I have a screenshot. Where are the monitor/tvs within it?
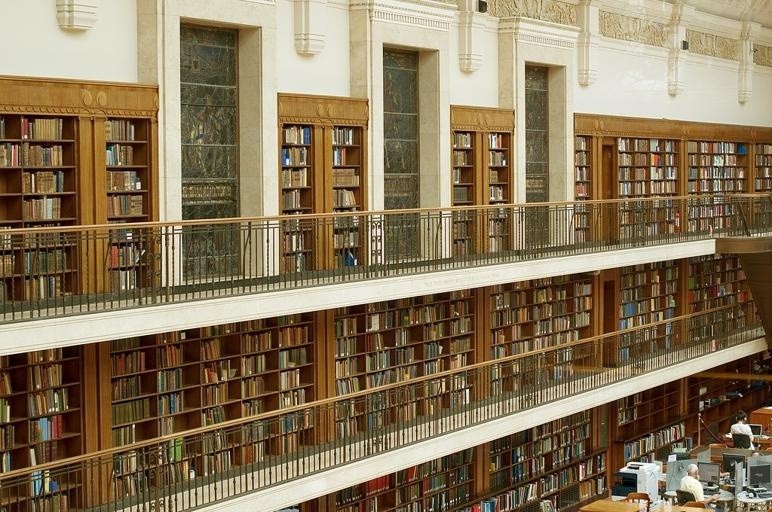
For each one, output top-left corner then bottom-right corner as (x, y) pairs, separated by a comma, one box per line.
(750, 464), (771, 485)
(611, 460), (657, 504)
(723, 453), (745, 479)
(697, 462), (720, 484)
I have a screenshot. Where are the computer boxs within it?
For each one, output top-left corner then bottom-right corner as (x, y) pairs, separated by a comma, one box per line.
(703, 486), (720, 494)
(691, 446), (711, 465)
(747, 487), (768, 497)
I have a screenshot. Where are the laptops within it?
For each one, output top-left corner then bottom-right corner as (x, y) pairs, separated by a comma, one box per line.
(749, 424), (762, 437)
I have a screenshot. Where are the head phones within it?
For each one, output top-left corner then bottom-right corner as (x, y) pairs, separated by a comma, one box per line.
(678, 463), (685, 471)
(735, 414), (747, 423)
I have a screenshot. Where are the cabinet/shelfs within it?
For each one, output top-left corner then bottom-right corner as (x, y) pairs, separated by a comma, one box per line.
(278, 107), (370, 276)
(574, 113), (772, 245)
(0, 109), (158, 313)
(1, 254), (771, 511)
(449, 105), (515, 258)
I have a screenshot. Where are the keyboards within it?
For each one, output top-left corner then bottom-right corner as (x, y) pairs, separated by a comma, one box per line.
(758, 493), (772, 498)
